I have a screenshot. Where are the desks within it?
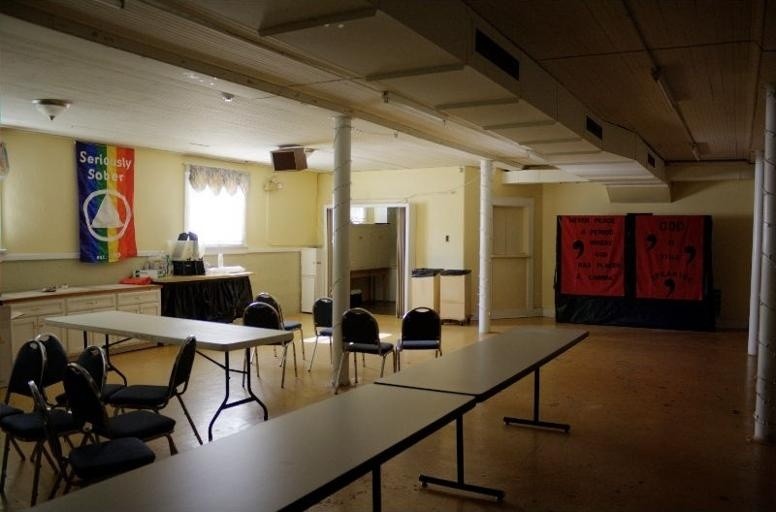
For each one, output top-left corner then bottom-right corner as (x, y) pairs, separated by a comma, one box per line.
(152, 271), (255, 324)
(348, 266), (391, 310)
(15, 384), (479, 512)
(373, 325), (589, 507)
(43, 310), (294, 443)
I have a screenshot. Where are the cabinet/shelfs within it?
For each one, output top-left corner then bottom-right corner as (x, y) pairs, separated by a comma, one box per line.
(0, 292), (67, 369)
(65, 287), (116, 360)
(115, 283), (163, 357)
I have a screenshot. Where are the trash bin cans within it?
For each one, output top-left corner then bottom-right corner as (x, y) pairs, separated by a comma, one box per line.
(350, 289), (362, 308)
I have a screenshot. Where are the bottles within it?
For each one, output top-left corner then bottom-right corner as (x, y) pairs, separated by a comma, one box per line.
(217, 253), (224, 269)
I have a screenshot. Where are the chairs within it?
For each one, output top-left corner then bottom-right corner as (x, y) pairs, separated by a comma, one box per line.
(331, 306), (396, 396)
(306, 297), (366, 374)
(2, 330), (200, 512)
(240, 301), (299, 389)
(250, 292), (306, 365)
(395, 306), (443, 373)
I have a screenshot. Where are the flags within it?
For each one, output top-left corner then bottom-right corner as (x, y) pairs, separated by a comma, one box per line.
(74, 141), (138, 264)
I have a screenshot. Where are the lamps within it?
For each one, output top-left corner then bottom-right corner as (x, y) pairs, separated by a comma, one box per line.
(31, 99), (69, 119)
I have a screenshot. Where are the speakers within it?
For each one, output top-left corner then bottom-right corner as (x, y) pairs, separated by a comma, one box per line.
(270, 147), (308, 171)
